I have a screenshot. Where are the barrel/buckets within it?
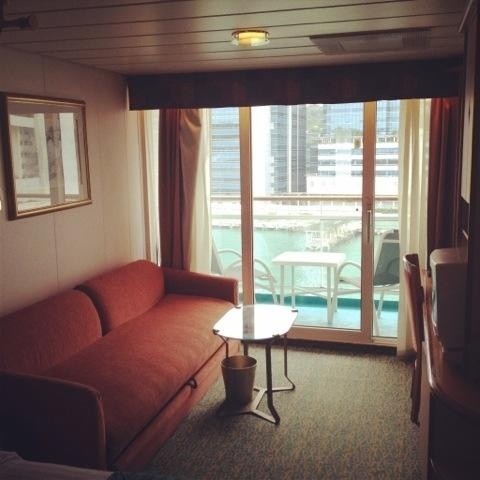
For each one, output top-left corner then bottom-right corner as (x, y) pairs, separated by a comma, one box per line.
(220, 355), (257, 403)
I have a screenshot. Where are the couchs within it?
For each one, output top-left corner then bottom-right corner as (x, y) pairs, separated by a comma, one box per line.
(0, 259), (242, 478)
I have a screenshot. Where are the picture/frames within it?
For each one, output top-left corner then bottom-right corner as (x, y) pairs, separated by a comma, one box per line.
(0, 92), (92, 221)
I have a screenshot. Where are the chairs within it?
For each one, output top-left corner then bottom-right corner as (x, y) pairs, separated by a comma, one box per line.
(331, 230), (401, 337)
(402, 254), (425, 424)
(218, 249), (278, 306)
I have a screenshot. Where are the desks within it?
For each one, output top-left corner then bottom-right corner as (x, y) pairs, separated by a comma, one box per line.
(271, 251), (346, 327)
(212, 303), (298, 424)
